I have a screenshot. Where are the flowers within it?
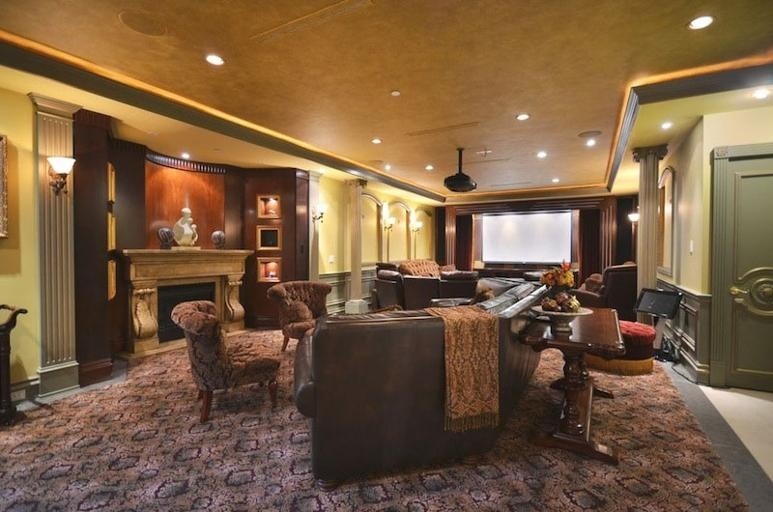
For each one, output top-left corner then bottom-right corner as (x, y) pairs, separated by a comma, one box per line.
(540, 259), (575, 288)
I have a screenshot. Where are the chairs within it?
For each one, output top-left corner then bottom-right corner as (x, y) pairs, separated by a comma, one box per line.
(266, 281), (332, 351)
(568, 261), (637, 322)
(170, 301), (282, 424)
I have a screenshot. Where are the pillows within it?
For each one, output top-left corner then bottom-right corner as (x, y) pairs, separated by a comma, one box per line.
(290, 300), (313, 322)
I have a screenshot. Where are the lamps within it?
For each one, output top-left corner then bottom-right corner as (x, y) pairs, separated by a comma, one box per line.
(412, 221), (423, 233)
(312, 205), (329, 223)
(628, 213), (640, 225)
(47, 157), (76, 195)
(384, 218), (396, 231)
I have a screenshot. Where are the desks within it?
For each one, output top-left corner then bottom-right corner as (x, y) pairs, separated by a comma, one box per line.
(520, 307), (627, 465)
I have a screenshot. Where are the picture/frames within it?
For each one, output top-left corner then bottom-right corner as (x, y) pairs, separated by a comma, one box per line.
(256, 192), (282, 282)
(0, 135), (8, 239)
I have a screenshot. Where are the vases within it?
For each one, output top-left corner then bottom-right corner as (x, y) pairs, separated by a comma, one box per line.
(551, 284), (566, 299)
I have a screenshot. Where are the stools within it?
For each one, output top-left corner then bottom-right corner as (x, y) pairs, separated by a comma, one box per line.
(584, 319), (656, 375)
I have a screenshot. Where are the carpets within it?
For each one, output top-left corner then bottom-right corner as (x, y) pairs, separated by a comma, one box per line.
(0, 328), (748, 512)
